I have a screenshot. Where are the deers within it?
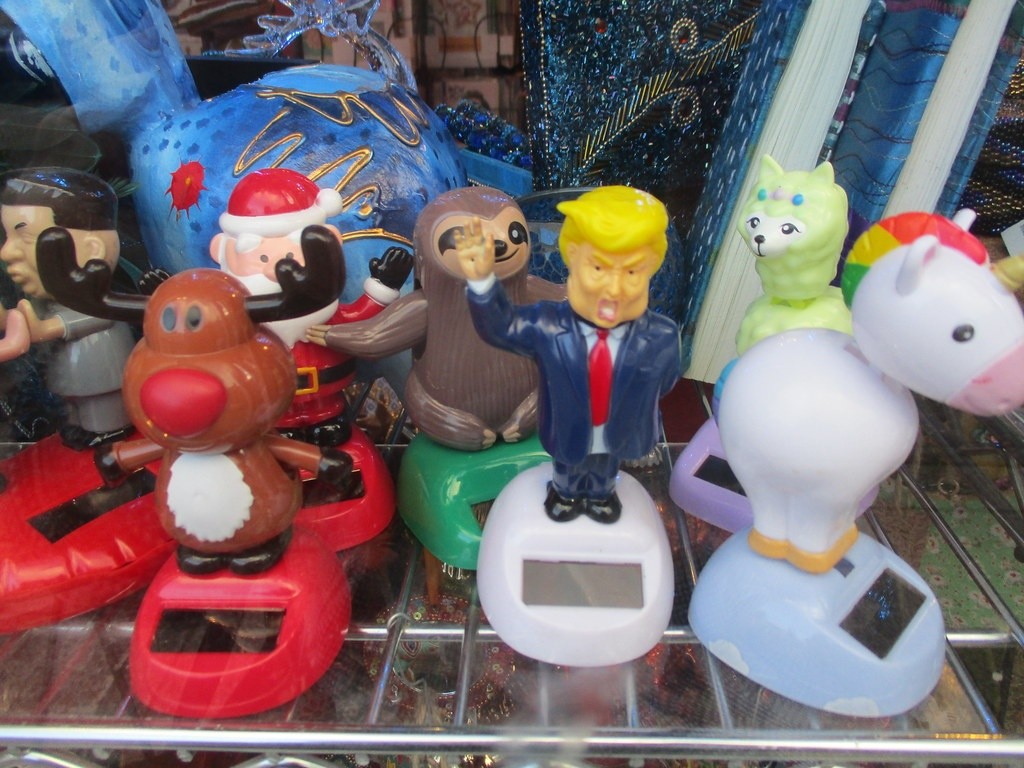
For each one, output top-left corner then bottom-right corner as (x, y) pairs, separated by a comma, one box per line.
(35, 224), (353, 571)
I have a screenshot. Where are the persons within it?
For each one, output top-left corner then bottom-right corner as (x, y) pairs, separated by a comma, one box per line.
(0, 165), (134, 450)
(210, 168), (413, 444)
(452, 186), (682, 525)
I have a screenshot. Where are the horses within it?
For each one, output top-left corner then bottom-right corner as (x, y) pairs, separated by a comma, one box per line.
(711, 208), (1024, 570)
